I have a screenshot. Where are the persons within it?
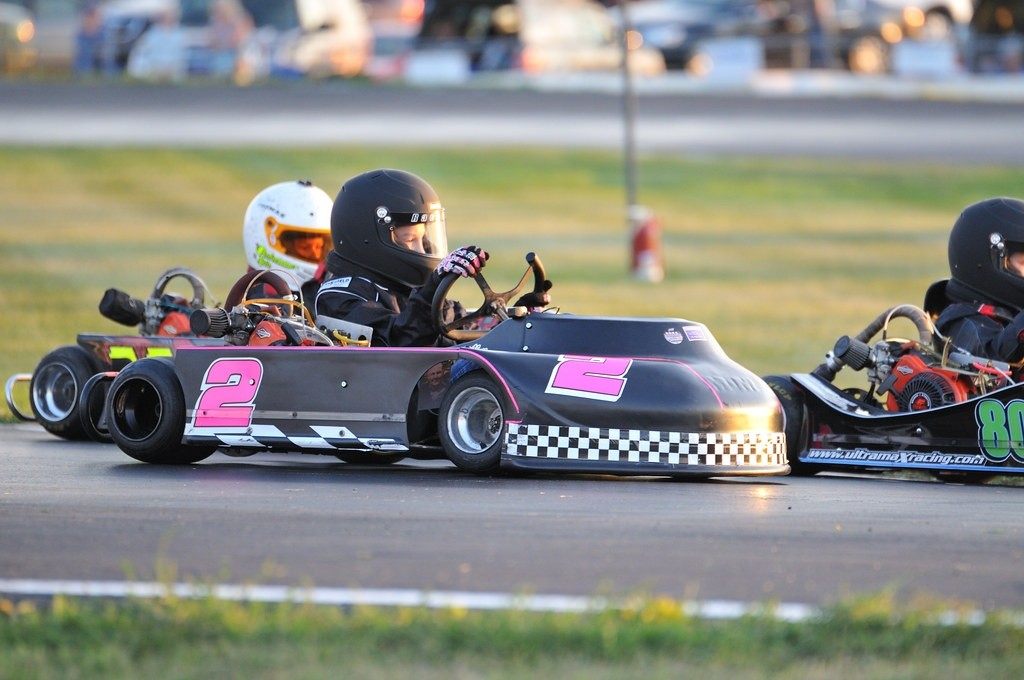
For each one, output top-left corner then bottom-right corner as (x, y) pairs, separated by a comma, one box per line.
(934, 198), (1024, 366)
(315, 169), (572, 347)
(225, 179), (332, 324)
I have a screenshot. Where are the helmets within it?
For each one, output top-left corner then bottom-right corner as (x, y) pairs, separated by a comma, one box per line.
(326, 168), (445, 287)
(243, 181), (333, 292)
(948, 198), (1024, 312)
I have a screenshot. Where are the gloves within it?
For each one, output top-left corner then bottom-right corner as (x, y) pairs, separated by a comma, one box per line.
(520, 279), (553, 313)
(427, 244), (491, 281)
